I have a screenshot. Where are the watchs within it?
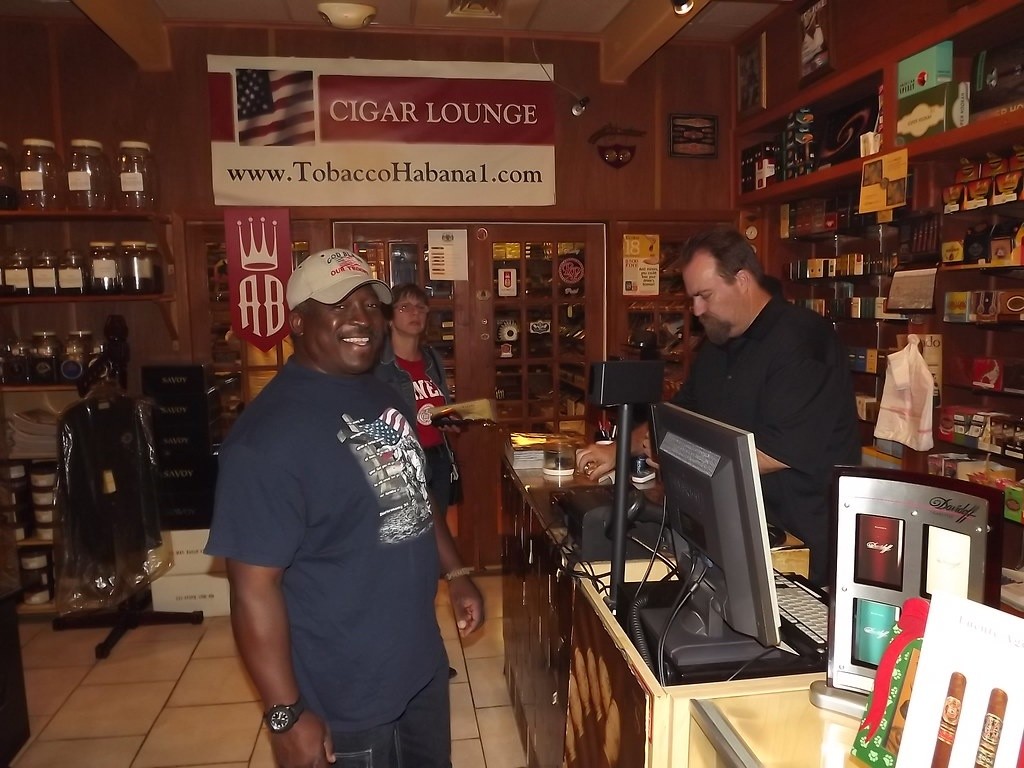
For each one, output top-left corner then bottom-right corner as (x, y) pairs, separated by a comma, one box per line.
(263, 696), (305, 734)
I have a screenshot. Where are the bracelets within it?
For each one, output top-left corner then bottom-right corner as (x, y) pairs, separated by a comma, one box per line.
(443, 567), (474, 581)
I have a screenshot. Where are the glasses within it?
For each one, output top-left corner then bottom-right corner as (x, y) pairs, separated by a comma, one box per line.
(400, 303), (430, 313)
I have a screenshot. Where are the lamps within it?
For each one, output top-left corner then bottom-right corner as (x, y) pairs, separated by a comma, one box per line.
(317, 3), (376, 31)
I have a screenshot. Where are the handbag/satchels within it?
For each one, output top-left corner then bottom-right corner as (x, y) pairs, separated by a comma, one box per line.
(442, 442), (463, 507)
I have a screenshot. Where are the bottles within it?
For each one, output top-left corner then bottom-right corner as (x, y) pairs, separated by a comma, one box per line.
(0, 142), (17, 210)
(29, 332), (63, 356)
(115, 141), (158, 211)
(119, 240), (152, 294)
(58, 250), (89, 295)
(5, 337), (30, 356)
(32, 250), (59, 294)
(146, 243), (165, 294)
(4, 246), (34, 295)
(65, 330), (94, 364)
(67, 139), (113, 210)
(87, 242), (122, 293)
(16, 138), (64, 210)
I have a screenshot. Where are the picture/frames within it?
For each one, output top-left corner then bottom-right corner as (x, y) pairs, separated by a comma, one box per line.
(735, 29), (768, 121)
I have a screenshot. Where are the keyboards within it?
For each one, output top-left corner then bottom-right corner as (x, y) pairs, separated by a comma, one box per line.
(771, 568), (830, 653)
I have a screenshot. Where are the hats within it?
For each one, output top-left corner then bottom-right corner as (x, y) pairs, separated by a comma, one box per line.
(287, 248), (394, 312)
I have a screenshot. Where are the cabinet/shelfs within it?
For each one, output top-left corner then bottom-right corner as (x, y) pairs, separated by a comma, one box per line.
(728, 1), (1023, 523)
(0, 208), (174, 611)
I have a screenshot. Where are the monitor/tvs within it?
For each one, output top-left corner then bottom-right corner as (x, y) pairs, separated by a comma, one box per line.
(638, 400), (780, 666)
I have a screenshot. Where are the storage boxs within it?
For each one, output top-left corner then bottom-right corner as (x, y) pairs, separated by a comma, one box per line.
(148, 529), (228, 576)
(155, 573), (230, 615)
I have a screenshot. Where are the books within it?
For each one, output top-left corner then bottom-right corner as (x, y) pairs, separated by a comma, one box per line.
(8, 408), (57, 458)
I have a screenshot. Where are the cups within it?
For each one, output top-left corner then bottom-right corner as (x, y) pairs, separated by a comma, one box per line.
(596, 440), (615, 445)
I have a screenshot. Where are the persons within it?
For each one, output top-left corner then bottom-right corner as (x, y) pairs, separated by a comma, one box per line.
(576, 223), (861, 593)
(202, 248), (485, 768)
(381, 283), (463, 519)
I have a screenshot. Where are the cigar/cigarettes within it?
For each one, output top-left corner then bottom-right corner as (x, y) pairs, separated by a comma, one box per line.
(931, 672), (967, 768)
(974, 688), (1008, 768)
